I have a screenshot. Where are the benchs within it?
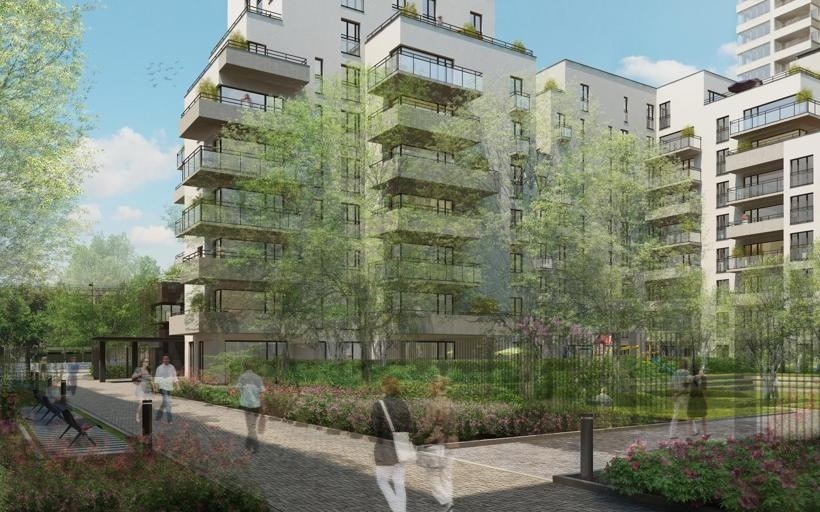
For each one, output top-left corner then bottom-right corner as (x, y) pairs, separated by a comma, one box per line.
(40, 395), (69, 426)
(59, 408), (104, 447)
(31, 388), (47, 414)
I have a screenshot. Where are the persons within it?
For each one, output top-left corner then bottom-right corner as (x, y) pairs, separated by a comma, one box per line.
(669, 359), (702, 440)
(367, 377), (411, 511)
(413, 374), (456, 509)
(67, 357), (80, 396)
(673, 363), (712, 439)
(154, 355), (180, 427)
(132, 357), (155, 425)
(237, 364), (265, 456)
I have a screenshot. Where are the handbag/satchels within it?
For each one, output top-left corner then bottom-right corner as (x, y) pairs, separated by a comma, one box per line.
(391, 431), (417, 465)
(258, 412), (266, 434)
(415, 443), (446, 468)
